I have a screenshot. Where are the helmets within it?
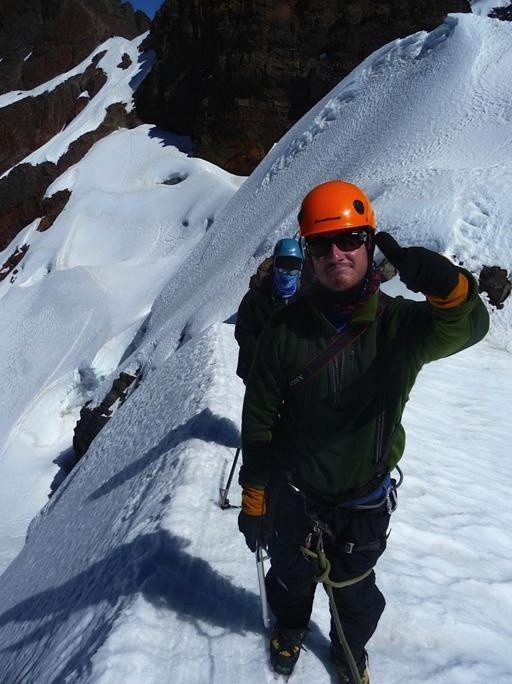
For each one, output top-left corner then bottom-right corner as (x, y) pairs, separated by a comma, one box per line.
(273, 238), (304, 261)
(298, 181), (376, 236)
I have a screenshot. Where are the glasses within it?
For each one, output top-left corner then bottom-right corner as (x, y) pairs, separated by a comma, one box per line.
(309, 231), (367, 256)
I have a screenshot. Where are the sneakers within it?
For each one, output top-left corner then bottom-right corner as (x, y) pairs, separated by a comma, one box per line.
(270, 624), (307, 675)
(330, 646), (369, 684)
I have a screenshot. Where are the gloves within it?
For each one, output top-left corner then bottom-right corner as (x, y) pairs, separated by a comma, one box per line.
(375, 230), (458, 298)
(237, 489), (277, 552)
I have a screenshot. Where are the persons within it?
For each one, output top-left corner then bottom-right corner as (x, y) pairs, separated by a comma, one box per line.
(234, 178), (491, 684)
(235, 237), (304, 384)
